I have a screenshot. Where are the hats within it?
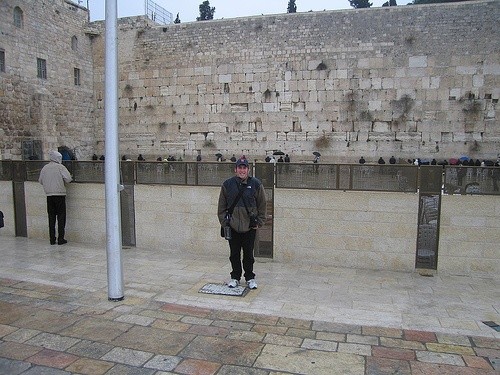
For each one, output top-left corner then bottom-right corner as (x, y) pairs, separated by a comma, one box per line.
(236, 158), (248, 167)
(240, 155), (246, 159)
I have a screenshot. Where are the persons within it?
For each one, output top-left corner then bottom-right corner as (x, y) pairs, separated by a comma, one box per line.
(278, 157), (283, 162)
(196, 152), (237, 162)
(217, 159), (267, 289)
(265, 156), (276, 166)
(407, 154), (500, 165)
(359, 156), (365, 164)
(390, 156), (396, 164)
(314, 155), (319, 163)
(378, 157), (385, 164)
(38, 151), (73, 245)
(92, 153), (183, 161)
(285, 154), (290, 162)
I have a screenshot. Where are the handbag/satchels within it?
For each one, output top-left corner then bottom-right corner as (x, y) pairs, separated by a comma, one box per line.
(221, 216), (230, 237)
(249, 216), (259, 229)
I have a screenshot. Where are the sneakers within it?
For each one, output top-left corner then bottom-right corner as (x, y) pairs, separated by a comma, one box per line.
(247, 279), (258, 289)
(228, 279), (240, 288)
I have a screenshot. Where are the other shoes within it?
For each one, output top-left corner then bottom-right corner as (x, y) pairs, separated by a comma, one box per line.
(50, 240), (56, 245)
(58, 239), (67, 245)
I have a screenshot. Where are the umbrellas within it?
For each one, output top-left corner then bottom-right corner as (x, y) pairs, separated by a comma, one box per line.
(313, 152), (321, 156)
(216, 153), (223, 157)
(273, 152), (285, 155)
